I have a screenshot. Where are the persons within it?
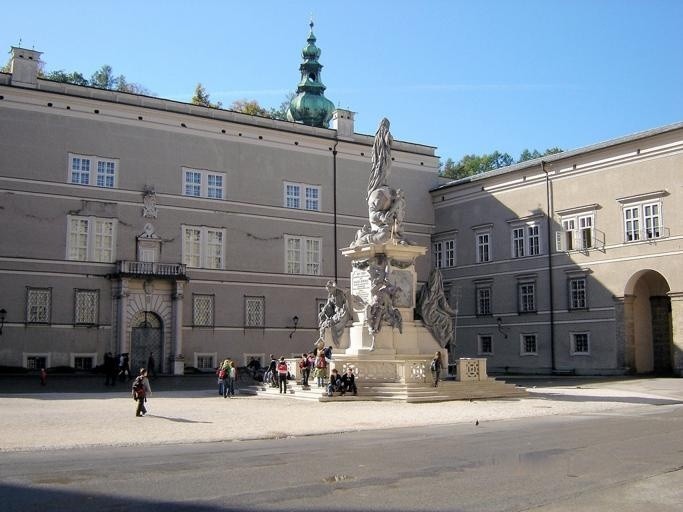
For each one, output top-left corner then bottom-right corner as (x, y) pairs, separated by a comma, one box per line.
(246, 354), (288, 394)
(105, 352), (132, 386)
(434, 351), (443, 387)
(147, 353), (158, 380)
(328, 368), (355, 396)
(41, 368), (46, 385)
(318, 117), (456, 346)
(216, 360), (237, 398)
(136, 368), (152, 416)
(300, 347), (333, 387)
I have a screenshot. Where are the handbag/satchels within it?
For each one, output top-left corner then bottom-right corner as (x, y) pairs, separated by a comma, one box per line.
(218, 379), (224, 384)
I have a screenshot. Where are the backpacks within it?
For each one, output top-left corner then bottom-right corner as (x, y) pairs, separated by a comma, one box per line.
(431, 359), (440, 371)
(132, 376), (146, 399)
(219, 368), (229, 379)
(278, 361), (287, 371)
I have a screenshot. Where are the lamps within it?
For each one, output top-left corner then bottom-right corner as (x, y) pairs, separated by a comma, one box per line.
(287, 316), (299, 337)
(0, 308), (6, 333)
(496, 316), (508, 341)
